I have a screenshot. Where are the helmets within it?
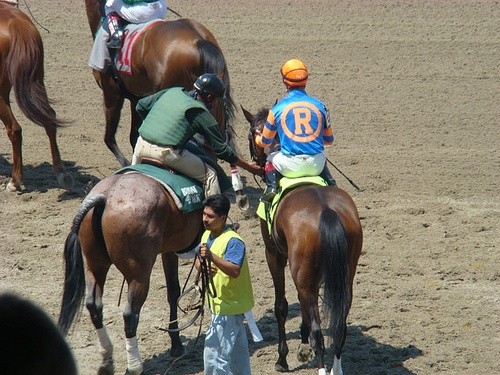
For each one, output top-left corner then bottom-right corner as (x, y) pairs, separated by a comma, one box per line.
(280, 59), (309, 87)
(193, 73), (225, 99)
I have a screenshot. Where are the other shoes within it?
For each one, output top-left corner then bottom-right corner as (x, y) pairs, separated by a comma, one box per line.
(227, 221), (240, 232)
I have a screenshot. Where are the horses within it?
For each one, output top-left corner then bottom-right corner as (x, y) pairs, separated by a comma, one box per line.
(238, 100), (363, 375)
(0, 0), (73, 192)
(58, 97), (232, 375)
(85, 1), (250, 211)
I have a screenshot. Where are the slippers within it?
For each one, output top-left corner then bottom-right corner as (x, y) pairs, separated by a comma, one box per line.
(318, 161), (336, 186)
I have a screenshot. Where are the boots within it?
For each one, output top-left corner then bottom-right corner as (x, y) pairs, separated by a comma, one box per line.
(261, 169), (280, 200)
(105, 11), (124, 49)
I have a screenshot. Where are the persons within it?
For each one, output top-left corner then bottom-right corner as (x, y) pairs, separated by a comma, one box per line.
(131, 74), (265, 230)
(195, 194), (254, 375)
(256, 58), (337, 204)
(89, 0), (167, 73)
